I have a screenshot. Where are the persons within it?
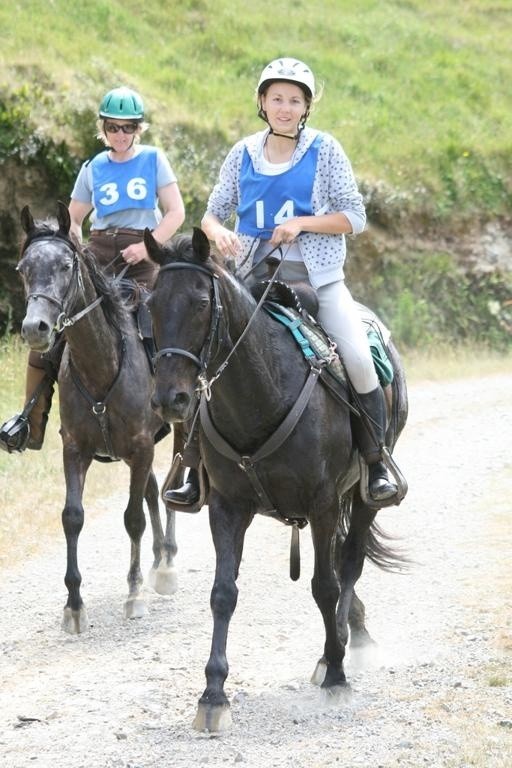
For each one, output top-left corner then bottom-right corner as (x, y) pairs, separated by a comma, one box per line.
(163, 57), (397, 505)
(12, 87), (185, 449)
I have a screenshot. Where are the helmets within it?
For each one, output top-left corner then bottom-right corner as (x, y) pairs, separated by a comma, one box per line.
(99, 87), (144, 119)
(256, 57), (314, 109)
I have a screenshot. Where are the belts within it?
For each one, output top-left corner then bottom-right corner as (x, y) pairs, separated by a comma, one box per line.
(91, 229), (143, 235)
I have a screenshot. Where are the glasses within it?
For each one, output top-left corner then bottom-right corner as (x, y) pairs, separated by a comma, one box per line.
(104, 123), (136, 134)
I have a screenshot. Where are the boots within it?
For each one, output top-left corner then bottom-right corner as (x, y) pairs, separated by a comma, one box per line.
(162, 399), (210, 504)
(356, 384), (398, 501)
(1, 365), (54, 450)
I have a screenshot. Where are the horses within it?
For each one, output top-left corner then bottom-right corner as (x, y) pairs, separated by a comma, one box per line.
(0, 200), (184, 635)
(143, 227), (408, 733)
